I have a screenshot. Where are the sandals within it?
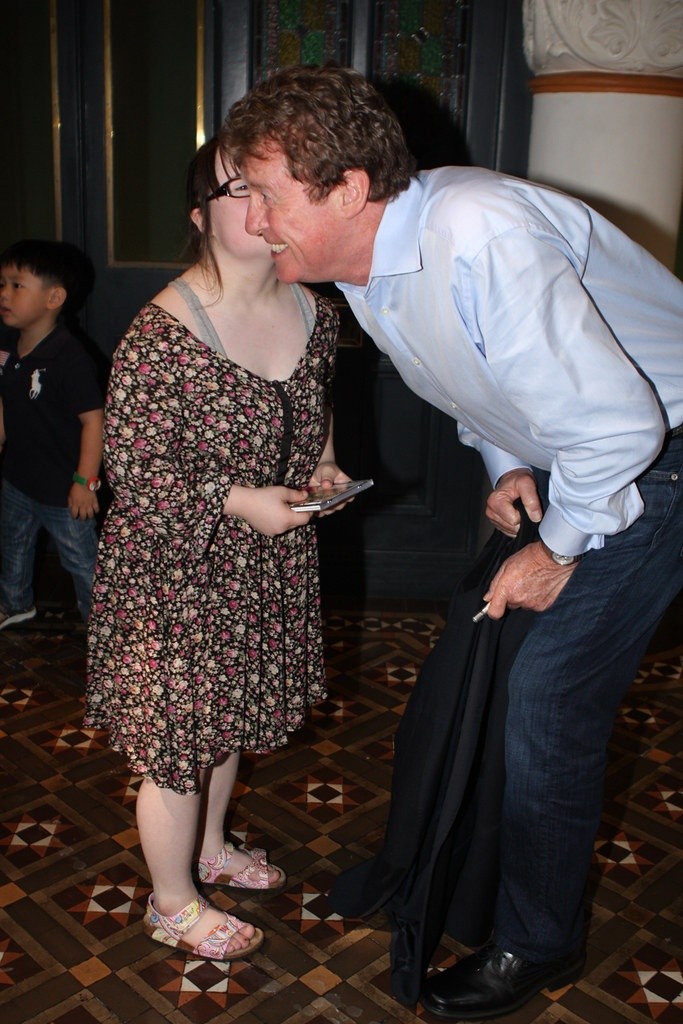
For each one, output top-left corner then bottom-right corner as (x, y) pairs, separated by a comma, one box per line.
(143, 893), (264, 960)
(0, 603), (36, 629)
(197, 842), (286, 889)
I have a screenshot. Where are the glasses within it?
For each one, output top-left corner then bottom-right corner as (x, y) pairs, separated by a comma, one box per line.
(205, 177), (251, 198)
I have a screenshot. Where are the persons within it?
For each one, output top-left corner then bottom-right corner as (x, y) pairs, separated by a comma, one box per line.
(81, 131), (356, 961)
(0, 241), (104, 630)
(219, 62), (683, 1024)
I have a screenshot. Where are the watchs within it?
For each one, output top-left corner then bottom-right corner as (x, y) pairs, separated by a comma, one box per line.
(540, 536), (587, 566)
(72, 472), (101, 492)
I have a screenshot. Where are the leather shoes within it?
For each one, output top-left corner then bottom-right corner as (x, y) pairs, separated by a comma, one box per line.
(420, 938), (586, 1022)
